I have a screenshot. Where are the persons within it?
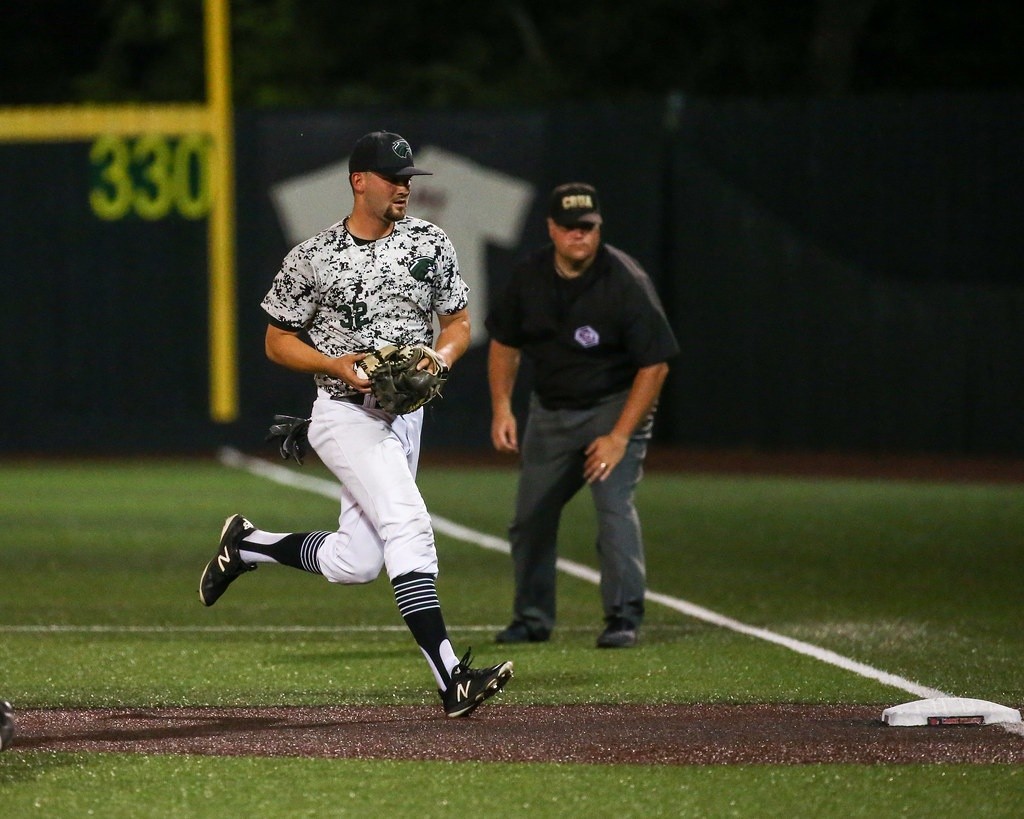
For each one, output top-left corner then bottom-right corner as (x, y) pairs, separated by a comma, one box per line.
(484, 183), (678, 647)
(198, 130), (514, 718)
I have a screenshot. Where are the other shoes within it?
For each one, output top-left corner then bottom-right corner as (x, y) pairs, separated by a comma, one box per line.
(496, 621), (551, 643)
(598, 616), (637, 647)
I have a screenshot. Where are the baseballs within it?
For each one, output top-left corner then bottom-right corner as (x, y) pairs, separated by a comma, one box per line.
(357, 366), (369, 380)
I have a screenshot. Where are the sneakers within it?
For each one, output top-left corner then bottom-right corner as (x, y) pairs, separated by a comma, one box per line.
(197, 514), (258, 607)
(438, 647), (514, 718)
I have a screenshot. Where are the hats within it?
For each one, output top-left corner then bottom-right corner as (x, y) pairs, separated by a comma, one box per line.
(349, 130), (434, 176)
(548, 184), (602, 228)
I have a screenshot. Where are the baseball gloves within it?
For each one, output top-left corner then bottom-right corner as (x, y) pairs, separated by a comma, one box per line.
(359, 343), (451, 417)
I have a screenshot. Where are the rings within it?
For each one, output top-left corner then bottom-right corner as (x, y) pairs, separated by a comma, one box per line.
(601, 462), (606, 468)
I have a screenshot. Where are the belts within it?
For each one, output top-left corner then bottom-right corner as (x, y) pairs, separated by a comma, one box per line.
(330, 393), (381, 408)
